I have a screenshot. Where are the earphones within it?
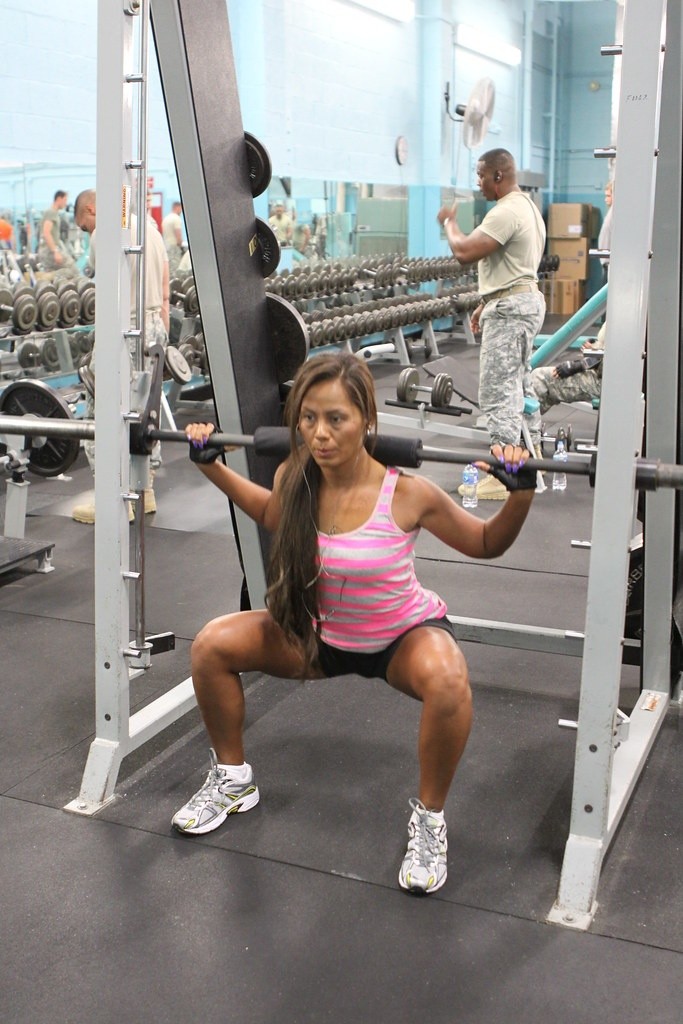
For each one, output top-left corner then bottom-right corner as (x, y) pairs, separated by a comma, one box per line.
(494, 174), (502, 184)
(366, 423), (371, 436)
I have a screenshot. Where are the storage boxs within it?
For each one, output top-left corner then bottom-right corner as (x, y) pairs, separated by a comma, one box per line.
(546, 237), (594, 281)
(537, 277), (587, 315)
(546, 201), (601, 241)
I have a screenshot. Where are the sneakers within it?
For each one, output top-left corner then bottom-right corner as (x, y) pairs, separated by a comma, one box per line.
(171, 764), (260, 834)
(127, 489), (157, 514)
(458, 474), (510, 500)
(73, 499), (134, 525)
(398, 804), (447, 893)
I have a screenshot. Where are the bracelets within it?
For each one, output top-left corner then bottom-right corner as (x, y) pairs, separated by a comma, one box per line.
(442, 217), (452, 226)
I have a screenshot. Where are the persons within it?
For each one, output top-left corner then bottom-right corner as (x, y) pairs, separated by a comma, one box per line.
(433, 150), (547, 500)
(596, 179), (609, 327)
(135, 190), (193, 270)
(518, 322), (605, 456)
(266, 201), (327, 263)
(2, 189), (74, 270)
(166, 353), (540, 893)
(73, 188), (162, 525)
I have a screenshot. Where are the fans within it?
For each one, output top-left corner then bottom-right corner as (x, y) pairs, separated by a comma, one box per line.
(456, 77), (496, 152)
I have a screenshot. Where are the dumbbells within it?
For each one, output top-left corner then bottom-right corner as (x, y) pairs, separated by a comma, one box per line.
(396, 368), (453, 409)
(540, 422), (574, 454)
(1, 253), (561, 386)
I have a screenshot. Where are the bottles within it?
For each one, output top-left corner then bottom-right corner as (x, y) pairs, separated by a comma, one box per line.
(551, 440), (567, 490)
(463, 462), (478, 508)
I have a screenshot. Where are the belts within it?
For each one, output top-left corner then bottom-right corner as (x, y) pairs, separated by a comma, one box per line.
(483, 284), (538, 303)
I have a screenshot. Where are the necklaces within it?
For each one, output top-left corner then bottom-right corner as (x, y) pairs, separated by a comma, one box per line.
(322, 445), (362, 535)
